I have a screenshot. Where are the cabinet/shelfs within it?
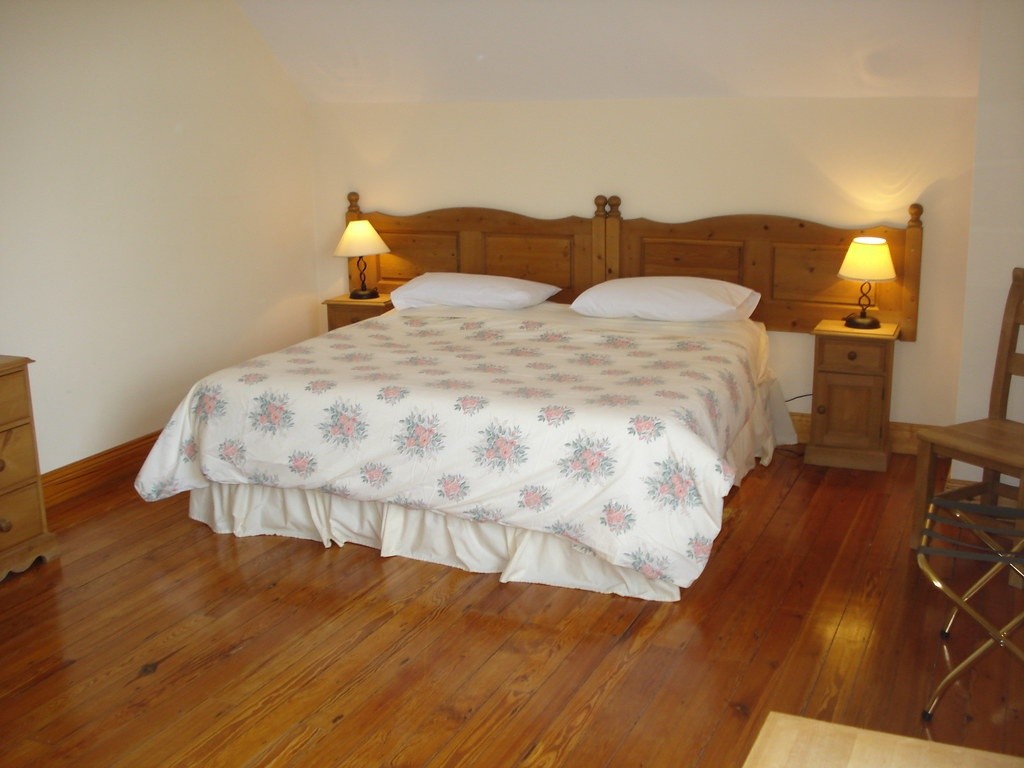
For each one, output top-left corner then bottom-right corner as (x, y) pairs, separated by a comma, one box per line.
(0, 354), (55, 585)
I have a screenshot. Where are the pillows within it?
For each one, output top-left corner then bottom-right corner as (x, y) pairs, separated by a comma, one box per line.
(571, 277), (762, 325)
(390, 271), (562, 311)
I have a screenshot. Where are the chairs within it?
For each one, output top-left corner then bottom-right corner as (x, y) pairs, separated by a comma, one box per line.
(905, 262), (1024, 590)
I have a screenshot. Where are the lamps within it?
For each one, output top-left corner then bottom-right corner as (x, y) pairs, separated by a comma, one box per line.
(334, 219), (390, 302)
(835, 237), (897, 331)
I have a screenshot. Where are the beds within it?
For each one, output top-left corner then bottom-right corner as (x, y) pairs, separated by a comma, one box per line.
(133, 184), (924, 603)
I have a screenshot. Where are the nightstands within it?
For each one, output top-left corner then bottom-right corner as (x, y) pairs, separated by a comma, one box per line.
(802, 317), (900, 474)
(322, 291), (393, 336)
